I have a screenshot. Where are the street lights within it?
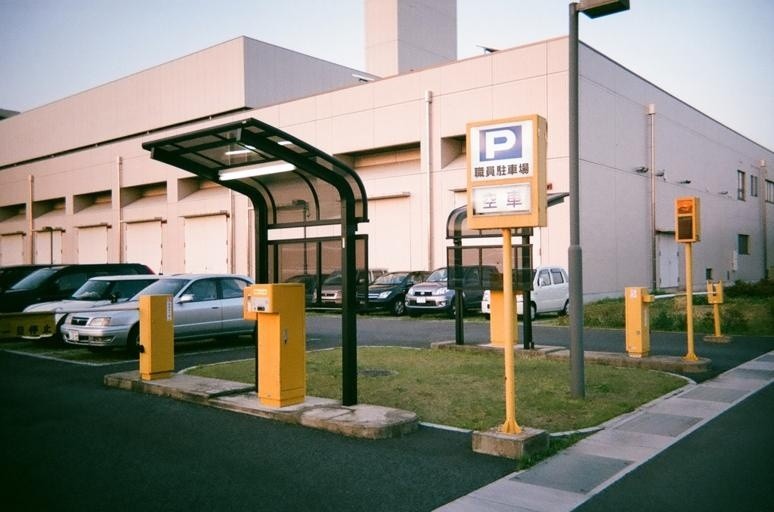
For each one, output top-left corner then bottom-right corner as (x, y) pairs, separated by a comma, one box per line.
(568, 0), (630, 398)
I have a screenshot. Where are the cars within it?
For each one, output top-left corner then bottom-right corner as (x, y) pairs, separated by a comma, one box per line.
(284, 265), (568, 321)
(1, 264), (256, 359)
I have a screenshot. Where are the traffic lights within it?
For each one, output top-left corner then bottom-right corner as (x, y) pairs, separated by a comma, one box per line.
(674, 197), (700, 243)
